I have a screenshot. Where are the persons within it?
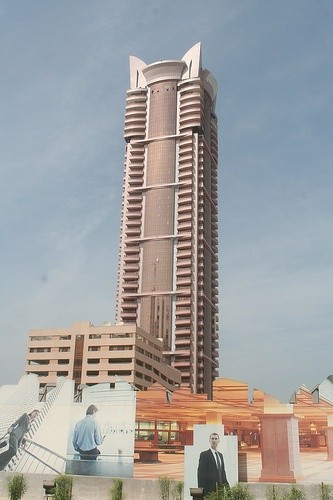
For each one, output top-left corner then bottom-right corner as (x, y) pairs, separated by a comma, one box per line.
(0, 410), (39, 471)
(197, 433), (231, 498)
(73, 404), (104, 460)
